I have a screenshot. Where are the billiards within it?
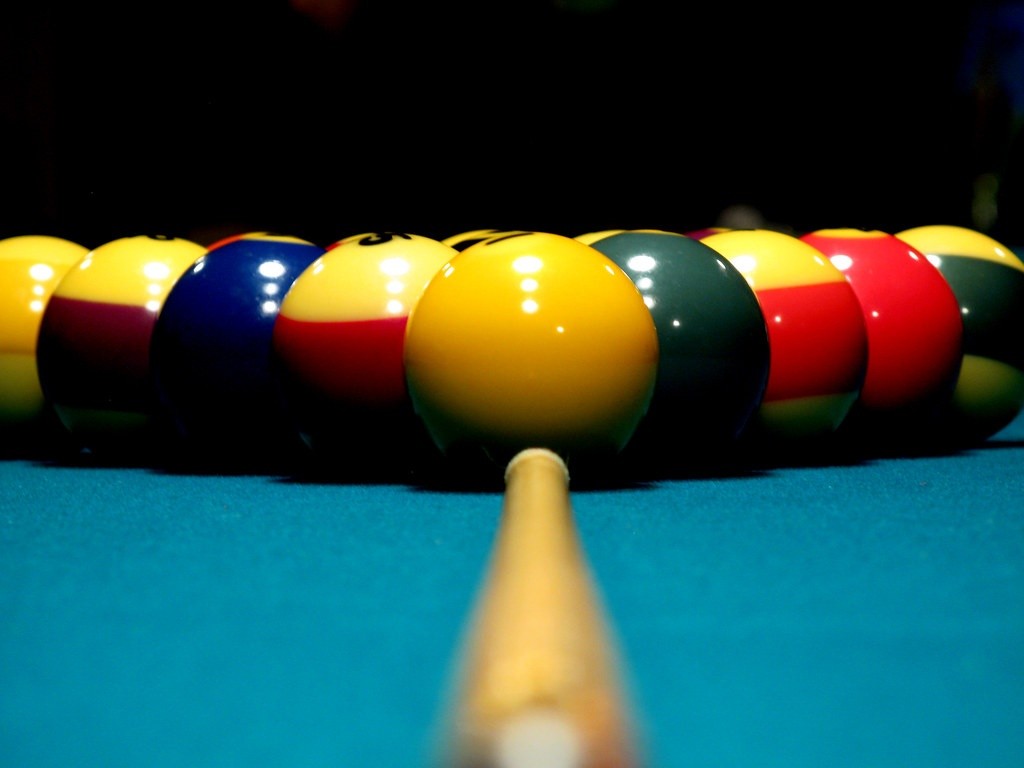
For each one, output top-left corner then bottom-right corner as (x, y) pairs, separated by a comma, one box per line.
(0, 224), (1022, 478)
(401, 230), (662, 488)
